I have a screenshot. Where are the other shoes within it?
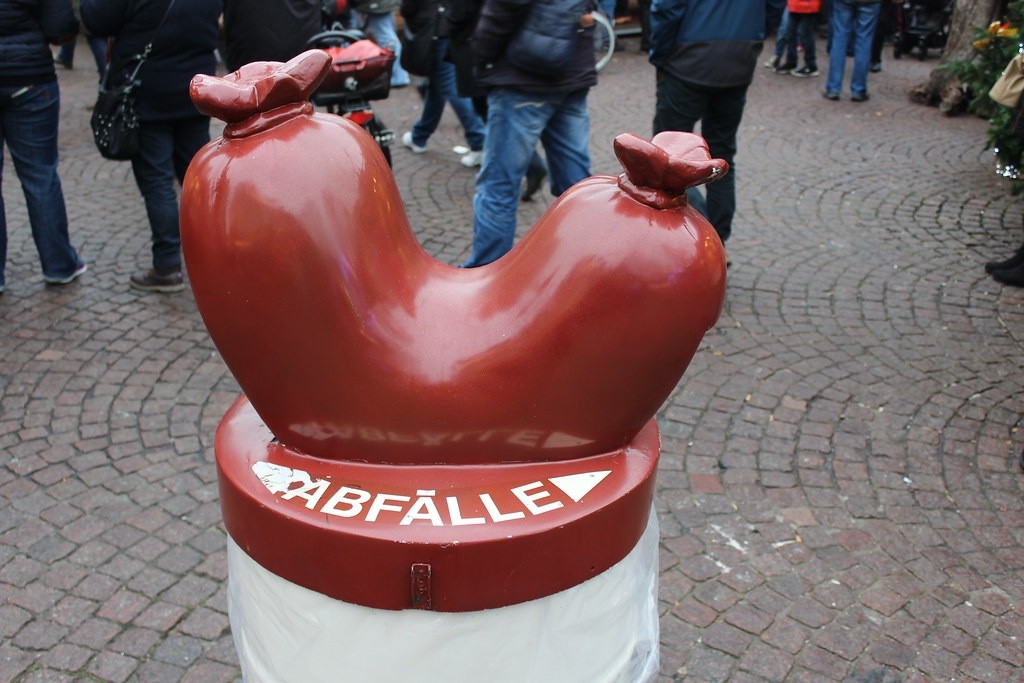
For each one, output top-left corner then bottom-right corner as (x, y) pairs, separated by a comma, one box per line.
(852, 92), (868, 101)
(821, 85), (838, 99)
(776, 61), (796, 74)
(523, 163), (548, 201)
(765, 53), (781, 69)
(872, 62), (880, 71)
(792, 65), (821, 78)
(53, 53), (72, 70)
(402, 129), (425, 152)
(602, 41), (626, 53)
(984, 243), (1024, 285)
(44, 263), (86, 284)
(460, 152), (485, 167)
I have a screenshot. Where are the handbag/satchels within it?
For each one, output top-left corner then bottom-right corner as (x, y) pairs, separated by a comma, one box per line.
(398, 22), (437, 77)
(988, 54), (1024, 106)
(89, 72), (137, 159)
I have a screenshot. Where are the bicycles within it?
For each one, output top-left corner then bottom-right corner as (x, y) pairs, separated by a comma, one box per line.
(305, 15), (396, 169)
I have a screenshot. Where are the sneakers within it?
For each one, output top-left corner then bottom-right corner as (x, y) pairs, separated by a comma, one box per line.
(129, 269), (183, 294)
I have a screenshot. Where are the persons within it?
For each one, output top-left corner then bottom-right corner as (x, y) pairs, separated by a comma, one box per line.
(0, 0), (889, 292)
(984, 48), (1024, 288)
